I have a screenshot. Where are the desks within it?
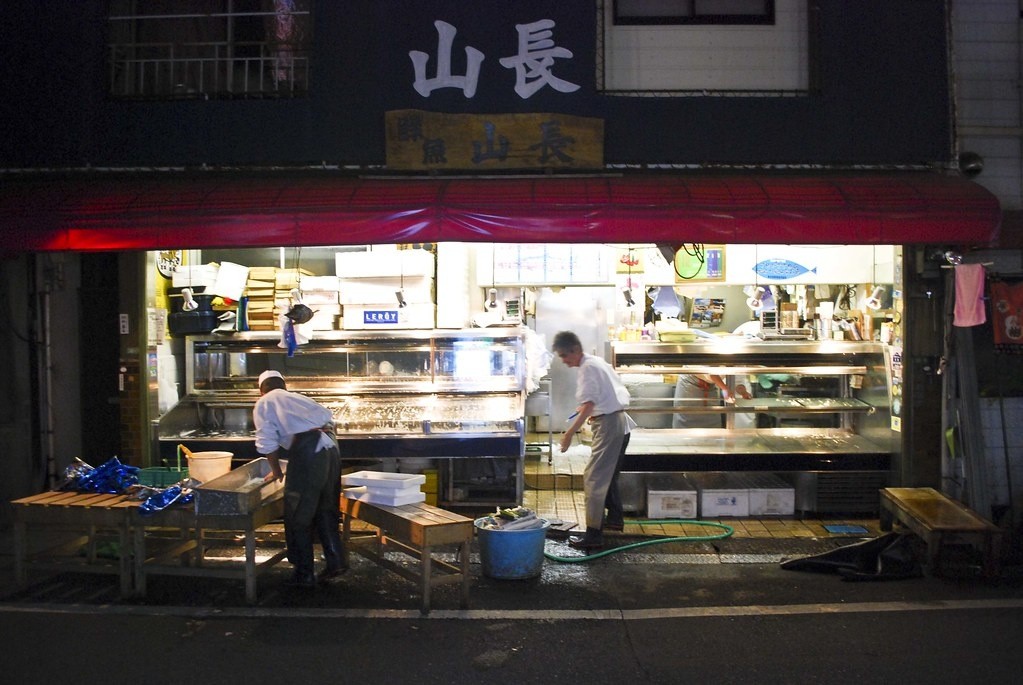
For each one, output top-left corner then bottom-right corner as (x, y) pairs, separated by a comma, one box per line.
(13, 469), (191, 600)
(334, 493), (474, 616)
(130, 492), (291, 604)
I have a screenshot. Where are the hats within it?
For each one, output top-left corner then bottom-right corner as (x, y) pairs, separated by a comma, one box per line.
(259, 370), (285, 388)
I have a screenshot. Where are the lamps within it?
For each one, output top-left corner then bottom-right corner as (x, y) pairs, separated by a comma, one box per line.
(622, 248), (641, 312)
(181, 253), (197, 312)
(865, 248), (886, 308)
(486, 242), (504, 313)
(746, 246), (766, 309)
(293, 251), (306, 308)
(395, 247), (409, 322)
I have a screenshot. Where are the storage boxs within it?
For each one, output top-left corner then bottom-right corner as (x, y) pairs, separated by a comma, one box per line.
(647, 473), (795, 520)
(246, 247), (436, 329)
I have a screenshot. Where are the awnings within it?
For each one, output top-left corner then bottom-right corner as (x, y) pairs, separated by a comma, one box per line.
(0, 172), (1005, 253)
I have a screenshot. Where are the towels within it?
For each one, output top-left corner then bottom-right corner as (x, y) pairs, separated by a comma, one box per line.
(954, 264), (986, 327)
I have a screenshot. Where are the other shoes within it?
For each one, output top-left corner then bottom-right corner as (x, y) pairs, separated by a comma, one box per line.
(604, 511), (624, 529)
(569, 526), (604, 547)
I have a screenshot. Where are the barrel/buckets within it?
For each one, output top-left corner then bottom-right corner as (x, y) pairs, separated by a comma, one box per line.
(474, 516), (551, 580)
(186, 451), (234, 484)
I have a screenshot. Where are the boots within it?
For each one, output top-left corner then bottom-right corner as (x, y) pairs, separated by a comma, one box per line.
(317, 520), (347, 579)
(283, 542), (318, 599)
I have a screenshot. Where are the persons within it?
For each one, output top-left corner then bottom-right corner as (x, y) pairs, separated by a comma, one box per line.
(253, 370), (348, 584)
(552, 330), (637, 549)
(672, 332), (753, 429)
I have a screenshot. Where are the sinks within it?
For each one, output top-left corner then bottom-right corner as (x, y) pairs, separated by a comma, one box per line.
(193, 456), (288, 514)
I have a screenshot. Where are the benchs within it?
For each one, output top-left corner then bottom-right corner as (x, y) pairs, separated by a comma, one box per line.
(879, 487), (998, 584)
